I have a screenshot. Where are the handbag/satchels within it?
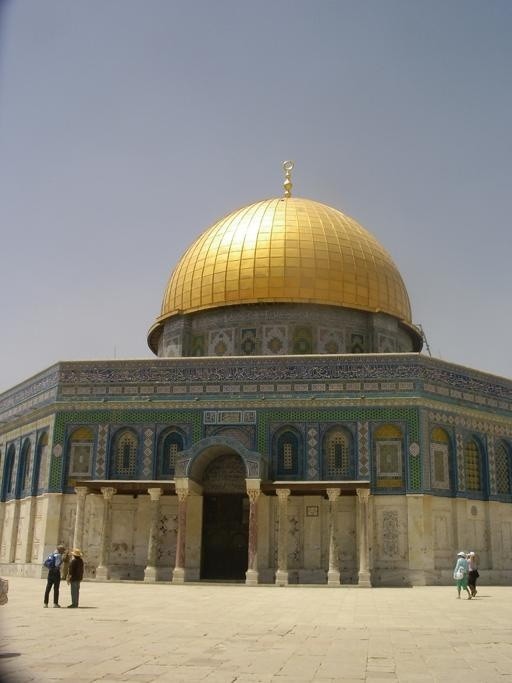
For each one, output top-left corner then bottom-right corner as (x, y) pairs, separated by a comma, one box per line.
(453, 572), (464, 579)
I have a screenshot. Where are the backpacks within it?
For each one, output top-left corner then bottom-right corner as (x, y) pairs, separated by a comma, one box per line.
(44, 553), (56, 568)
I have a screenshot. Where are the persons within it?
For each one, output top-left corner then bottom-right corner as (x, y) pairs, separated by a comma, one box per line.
(453, 551), (481, 600)
(66, 549), (84, 608)
(43, 544), (68, 608)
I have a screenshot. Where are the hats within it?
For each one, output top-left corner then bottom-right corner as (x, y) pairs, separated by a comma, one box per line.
(71, 548), (82, 556)
(57, 544), (66, 549)
(458, 552), (465, 557)
(468, 551), (476, 555)
